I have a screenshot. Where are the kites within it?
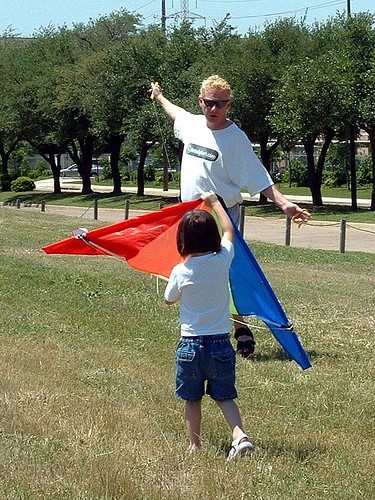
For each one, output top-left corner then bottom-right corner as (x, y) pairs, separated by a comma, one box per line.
(43, 194), (313, 372)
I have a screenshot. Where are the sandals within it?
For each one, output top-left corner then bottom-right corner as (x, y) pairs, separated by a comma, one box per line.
(234, 326), (256, 358)
(226, 434), (252, 463)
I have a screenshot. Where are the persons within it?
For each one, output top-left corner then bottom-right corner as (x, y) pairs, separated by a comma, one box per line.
(163, 190), (256, 463)
(151, 74), (310, 358)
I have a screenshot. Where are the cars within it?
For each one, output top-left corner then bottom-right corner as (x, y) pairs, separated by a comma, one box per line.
(60, 162), (105, 177)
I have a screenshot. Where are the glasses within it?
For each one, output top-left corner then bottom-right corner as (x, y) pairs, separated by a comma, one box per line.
(203, 99), (229, 108)
